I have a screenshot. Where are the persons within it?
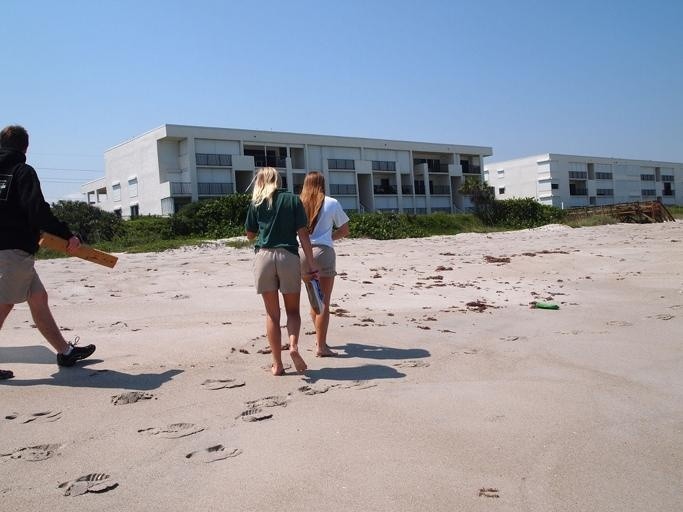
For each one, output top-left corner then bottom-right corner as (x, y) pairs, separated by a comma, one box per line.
(1, 124), (95, 380)
(243, 165), (321, 377)
(295, 170), (353, 358)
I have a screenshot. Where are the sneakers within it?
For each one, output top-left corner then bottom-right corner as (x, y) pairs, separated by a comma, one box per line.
(0, 369), (16, 380)
(55, 336), (97, 367)
(304, 278), (323, 316)
